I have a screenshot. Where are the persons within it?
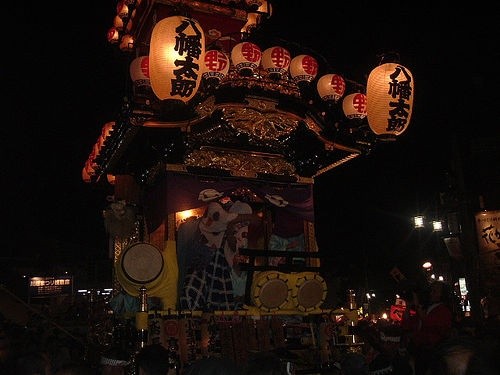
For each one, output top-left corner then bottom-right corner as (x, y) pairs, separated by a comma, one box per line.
(0, 324), (500, 375)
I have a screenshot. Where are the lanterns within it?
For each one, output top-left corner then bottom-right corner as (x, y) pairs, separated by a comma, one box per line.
(82, 0), (414, 182)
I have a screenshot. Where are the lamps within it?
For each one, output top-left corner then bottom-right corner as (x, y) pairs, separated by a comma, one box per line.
(410, 199), (444, 233)
